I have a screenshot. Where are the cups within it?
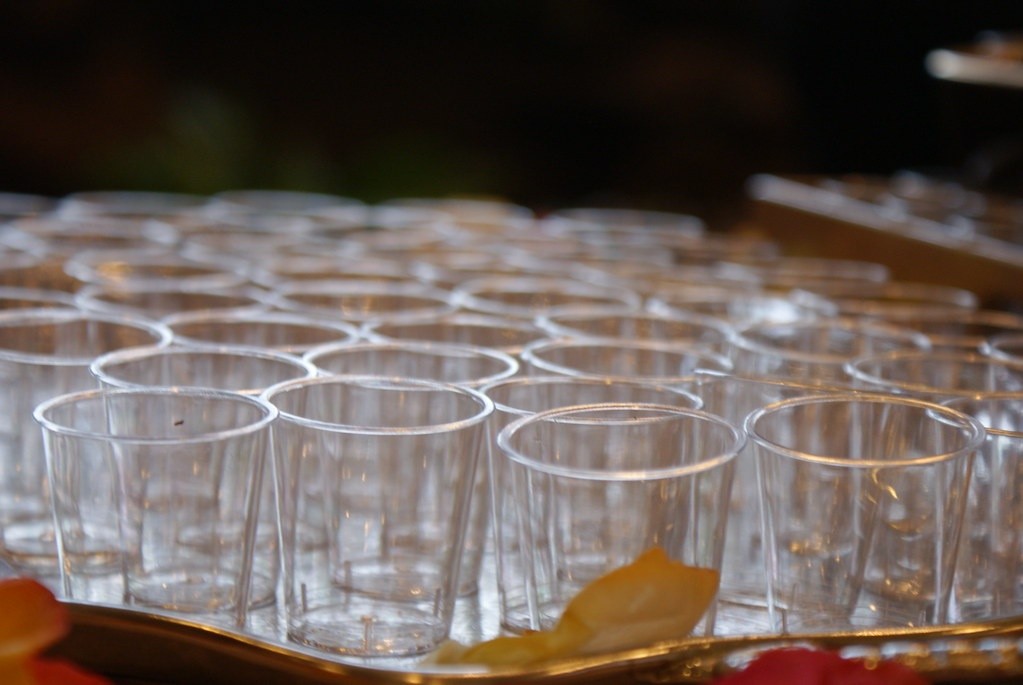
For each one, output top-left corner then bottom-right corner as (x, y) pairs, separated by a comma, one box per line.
(2, 191), (1022, 669)
